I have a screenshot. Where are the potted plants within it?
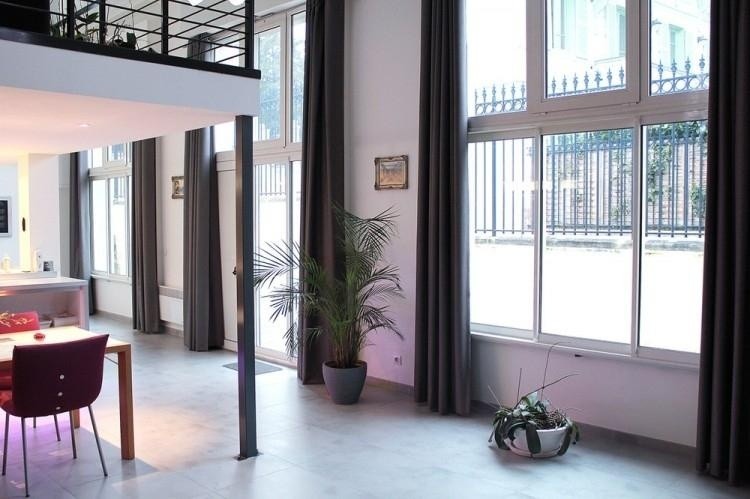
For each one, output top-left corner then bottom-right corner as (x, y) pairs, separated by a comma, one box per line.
(247, 196), (406, 405)
(487, 340), (585, 461)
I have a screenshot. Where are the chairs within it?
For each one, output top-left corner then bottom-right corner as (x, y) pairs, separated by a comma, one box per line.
(0, 309), (63, 443)
(0, 332), (108, 498)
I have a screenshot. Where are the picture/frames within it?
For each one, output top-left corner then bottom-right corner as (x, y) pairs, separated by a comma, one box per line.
(372, 154), (409, 191)
(171, 175), (184, 199)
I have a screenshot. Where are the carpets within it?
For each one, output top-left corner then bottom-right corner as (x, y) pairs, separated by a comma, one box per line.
(222, 360), (283, 375)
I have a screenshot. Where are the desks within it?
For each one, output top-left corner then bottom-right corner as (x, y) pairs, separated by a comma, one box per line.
(0, 275), (91, 331)
(0, 325), (135, 461)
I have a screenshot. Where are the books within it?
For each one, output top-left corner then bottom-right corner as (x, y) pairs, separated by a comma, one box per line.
(39, 311), (78, 330)
